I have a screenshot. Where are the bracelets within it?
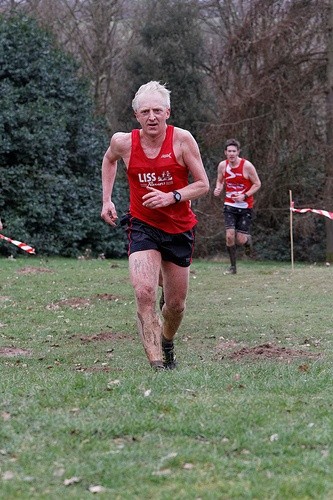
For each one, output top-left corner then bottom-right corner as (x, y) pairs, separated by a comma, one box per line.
(244, 193), (248, 199)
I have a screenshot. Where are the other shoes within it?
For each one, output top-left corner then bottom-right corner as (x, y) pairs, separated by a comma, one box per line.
(244, 241), (252, 256)
(223, 267), (236, 275)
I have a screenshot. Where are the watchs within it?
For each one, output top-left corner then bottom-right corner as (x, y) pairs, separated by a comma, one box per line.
(172, 190), (181, 205)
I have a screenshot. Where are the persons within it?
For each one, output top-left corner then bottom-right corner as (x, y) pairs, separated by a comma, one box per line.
(100, 81), (210, 377)
(214, 139), (262, 276)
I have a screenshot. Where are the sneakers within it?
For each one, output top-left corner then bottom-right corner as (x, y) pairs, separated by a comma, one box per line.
(161, 343), (176, 370)
(151, 366), (165, 373)
